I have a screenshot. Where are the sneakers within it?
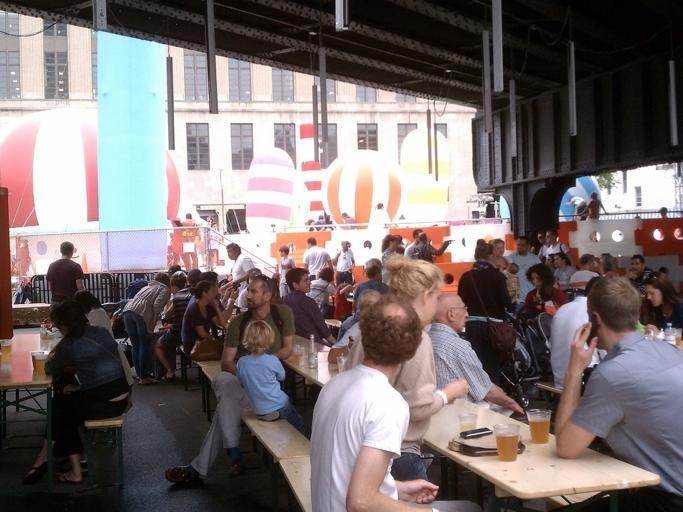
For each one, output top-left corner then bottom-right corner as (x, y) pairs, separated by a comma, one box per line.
(164, 466), (199, 482)
(140, 378), (160, 385)
(161, 371), (176, 380)
(228, 461), (246, 476)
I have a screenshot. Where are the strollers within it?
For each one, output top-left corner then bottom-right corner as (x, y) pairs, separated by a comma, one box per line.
(465, 317), (541, 407)
(525, 311), (584, 387)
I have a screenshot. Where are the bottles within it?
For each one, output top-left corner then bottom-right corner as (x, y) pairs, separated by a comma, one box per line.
(39, 322), (47, 340)
(663, 322), (676, 345)
(306, 335), (318, 370)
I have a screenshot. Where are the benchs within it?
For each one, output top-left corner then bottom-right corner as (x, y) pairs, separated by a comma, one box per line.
(81, 417), (129, 493)
(324, 318), (589, 398)
(192, 359), (312, 510)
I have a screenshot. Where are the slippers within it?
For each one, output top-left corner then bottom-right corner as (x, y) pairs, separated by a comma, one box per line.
(55, 472), (82, 484)
(23, 460), (48, 485)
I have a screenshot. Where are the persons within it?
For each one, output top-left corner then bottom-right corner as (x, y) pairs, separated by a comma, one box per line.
(46, 242), (85, 304)
(75, 290), (114, 338)
(121, 191), (682, 512)
(23, 301), (130, 485)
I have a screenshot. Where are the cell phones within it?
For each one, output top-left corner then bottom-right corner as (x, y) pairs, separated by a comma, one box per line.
(460, 427), (493, 439)
(586, 316), (598, 347)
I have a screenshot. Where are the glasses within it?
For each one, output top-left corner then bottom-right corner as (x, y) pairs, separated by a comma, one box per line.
(646, 272), (658, 280)
(255, 273), (269, 281)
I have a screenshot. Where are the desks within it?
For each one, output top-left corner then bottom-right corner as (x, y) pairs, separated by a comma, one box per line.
(1, 331), (64, 491)
(276, 335), (661, 510)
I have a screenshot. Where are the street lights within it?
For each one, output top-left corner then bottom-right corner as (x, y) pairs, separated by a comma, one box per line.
(218, 167), (226, 231)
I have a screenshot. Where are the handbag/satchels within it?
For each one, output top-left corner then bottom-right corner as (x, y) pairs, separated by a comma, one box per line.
(189, 335), (223, 361)
(481, 318), (516, 354)
(448, 431), (525, 457)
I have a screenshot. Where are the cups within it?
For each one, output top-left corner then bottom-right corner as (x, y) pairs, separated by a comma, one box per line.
(336, 356), (347, 374)
(0, 339), (12, 358)
(458, 412), (477, 431)
(492, 423), (523, 461)
(293, 340), (303, 356)
(29, 351), (47, 373)
(526, 408), (551, 444)
